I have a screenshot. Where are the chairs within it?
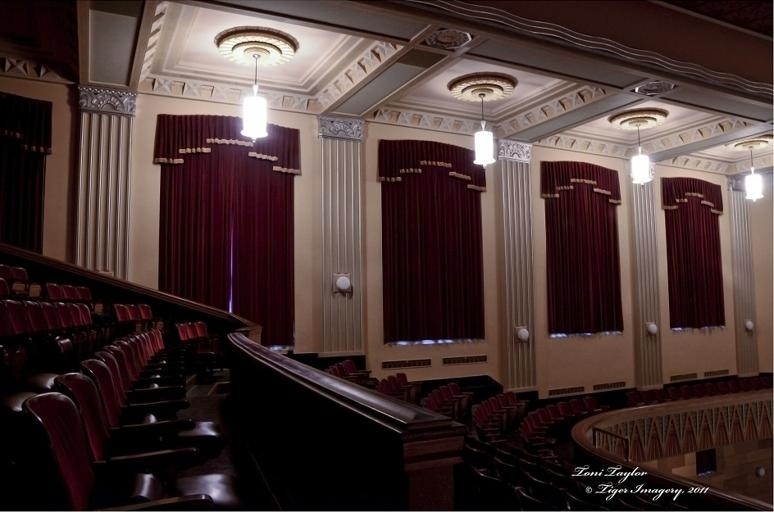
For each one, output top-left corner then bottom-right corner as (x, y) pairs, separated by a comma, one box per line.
(1, 266), (773, 511)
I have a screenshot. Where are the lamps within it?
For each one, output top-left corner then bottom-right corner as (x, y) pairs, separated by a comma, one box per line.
(735, 140), (768, 202)
(214, 27), (299, 139)
(608, 107), (670, 187)
(447, 72), (518, 169)
